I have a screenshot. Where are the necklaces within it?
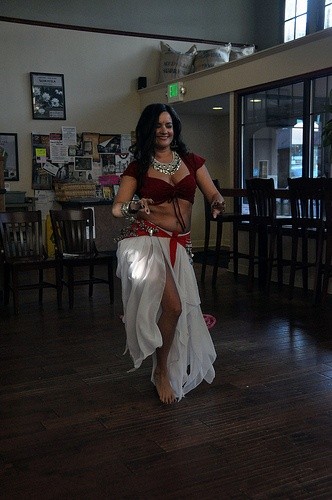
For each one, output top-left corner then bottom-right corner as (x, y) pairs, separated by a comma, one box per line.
(151, 151), (181, 176)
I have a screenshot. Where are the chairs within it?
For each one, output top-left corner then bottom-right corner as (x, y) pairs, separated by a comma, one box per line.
(199, 179), (249, 285)
(48, 209), (117, 310)
(0, 210), (59, 314)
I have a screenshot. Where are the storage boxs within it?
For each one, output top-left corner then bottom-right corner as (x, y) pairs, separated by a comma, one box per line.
(5, 191), (26, 204)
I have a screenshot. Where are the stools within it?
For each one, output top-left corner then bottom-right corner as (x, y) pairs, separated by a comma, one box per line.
(249, 177), (303, 299)
(288, 177), (332, 318)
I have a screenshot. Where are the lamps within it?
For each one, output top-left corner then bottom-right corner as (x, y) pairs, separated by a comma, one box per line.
(165, 81), (185, 103)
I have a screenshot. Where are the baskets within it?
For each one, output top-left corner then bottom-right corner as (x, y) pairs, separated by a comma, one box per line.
(53, 182), (96, 201)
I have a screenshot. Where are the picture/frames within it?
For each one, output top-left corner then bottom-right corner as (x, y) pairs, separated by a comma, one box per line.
(0, 133), (20, 182)
(30, 72), (66, 120)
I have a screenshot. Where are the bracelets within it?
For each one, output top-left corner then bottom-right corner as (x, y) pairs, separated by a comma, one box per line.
(121, 202), (138, 215)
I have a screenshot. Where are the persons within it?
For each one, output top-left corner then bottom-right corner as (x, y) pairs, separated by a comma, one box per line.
(111, 103), (226, 405)
